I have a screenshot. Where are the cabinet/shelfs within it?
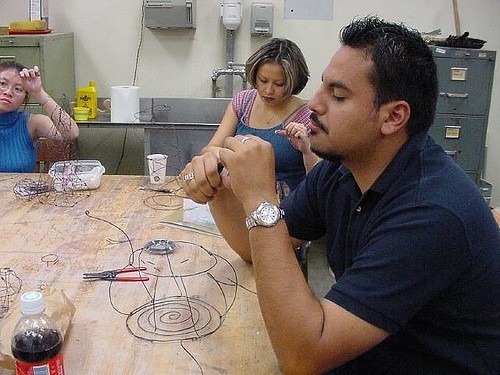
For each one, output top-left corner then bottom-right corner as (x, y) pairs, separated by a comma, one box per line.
(0, 32), (76, 115)
(429, 44), (496, 187)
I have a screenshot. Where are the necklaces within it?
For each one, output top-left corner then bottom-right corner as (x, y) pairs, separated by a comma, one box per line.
(265, 113), (276, 124)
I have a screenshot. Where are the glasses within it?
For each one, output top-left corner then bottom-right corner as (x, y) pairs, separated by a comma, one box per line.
(0, 81), (25, 96)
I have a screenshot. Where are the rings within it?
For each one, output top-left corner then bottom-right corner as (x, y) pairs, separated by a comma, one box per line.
(241, 137), (250, 144)
(184, 174), (195, 181)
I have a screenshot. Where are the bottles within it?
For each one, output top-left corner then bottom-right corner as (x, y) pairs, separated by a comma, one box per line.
(11, 292), (65, 375)
(77, 80), (97, 118)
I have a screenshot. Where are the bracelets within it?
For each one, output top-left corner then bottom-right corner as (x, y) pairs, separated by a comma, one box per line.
(39, 96), (50, 106)
(304, 157), (320, 171)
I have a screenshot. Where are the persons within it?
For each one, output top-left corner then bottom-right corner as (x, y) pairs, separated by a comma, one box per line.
(0, 61), (80, 173)
(200, 37), (323, 201)
(179, 13), (500, 375)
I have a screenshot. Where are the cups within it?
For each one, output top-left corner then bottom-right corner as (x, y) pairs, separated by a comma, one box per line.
(147, 154), (168, 184)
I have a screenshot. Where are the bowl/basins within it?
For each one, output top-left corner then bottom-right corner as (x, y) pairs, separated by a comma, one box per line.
(49, 160), (105, 192)
(74, 107), (90, 120)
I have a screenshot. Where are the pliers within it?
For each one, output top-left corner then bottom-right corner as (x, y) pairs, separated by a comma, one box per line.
(80, 266), (149, 282)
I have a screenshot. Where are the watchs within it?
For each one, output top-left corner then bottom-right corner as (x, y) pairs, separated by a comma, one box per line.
(245, 202), (286, 230)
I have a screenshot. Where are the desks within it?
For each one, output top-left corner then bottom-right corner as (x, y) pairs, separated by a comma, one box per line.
(0, 172), (280, 375)
